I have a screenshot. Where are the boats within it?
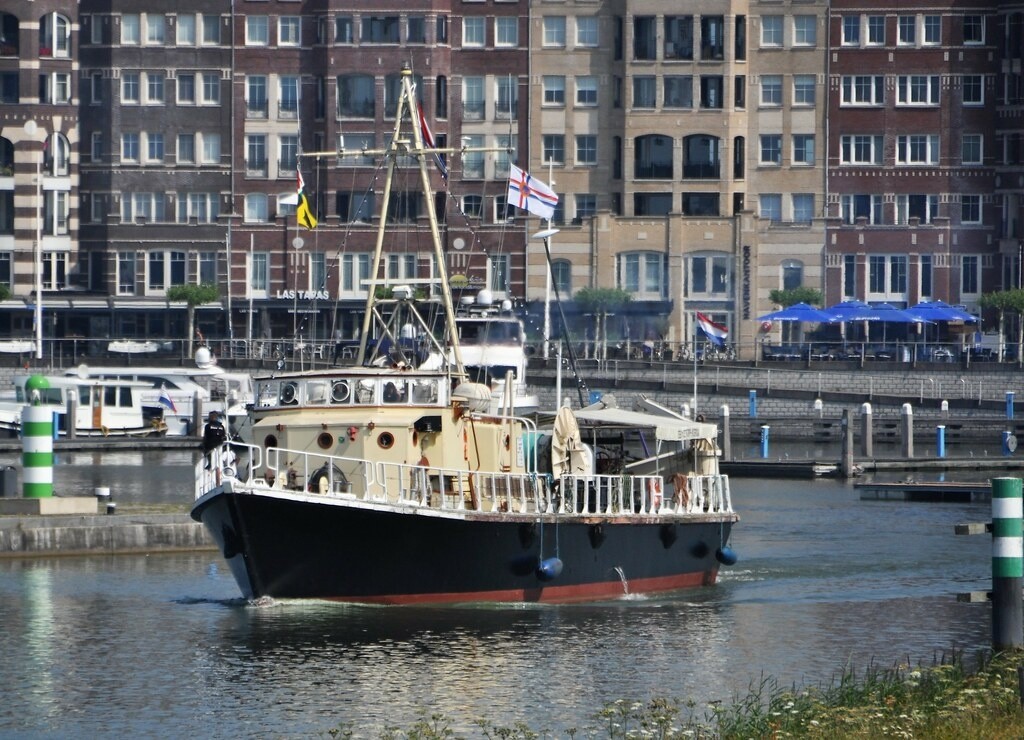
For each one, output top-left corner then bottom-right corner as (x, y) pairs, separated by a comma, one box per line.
(1, 343), (253, 439)
(305, 258), (541, 418)
(186, 1), (742, 608)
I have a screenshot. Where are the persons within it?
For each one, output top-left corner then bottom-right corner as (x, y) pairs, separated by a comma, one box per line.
(202, 409), (226, 469)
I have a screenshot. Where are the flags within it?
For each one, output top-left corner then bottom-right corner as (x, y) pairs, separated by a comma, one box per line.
(156, 382), (178, 416)
(508, 163), (559, 220)
(417, 100), (448, 178)
(294, 161), (320, 230)
(694, 309), (728, 349)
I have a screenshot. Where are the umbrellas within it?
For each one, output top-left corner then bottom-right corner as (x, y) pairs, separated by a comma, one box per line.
(756, 297), (986, 361)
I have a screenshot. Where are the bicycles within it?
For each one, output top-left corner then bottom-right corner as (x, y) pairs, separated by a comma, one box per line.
(676, 341), (737, 362)
